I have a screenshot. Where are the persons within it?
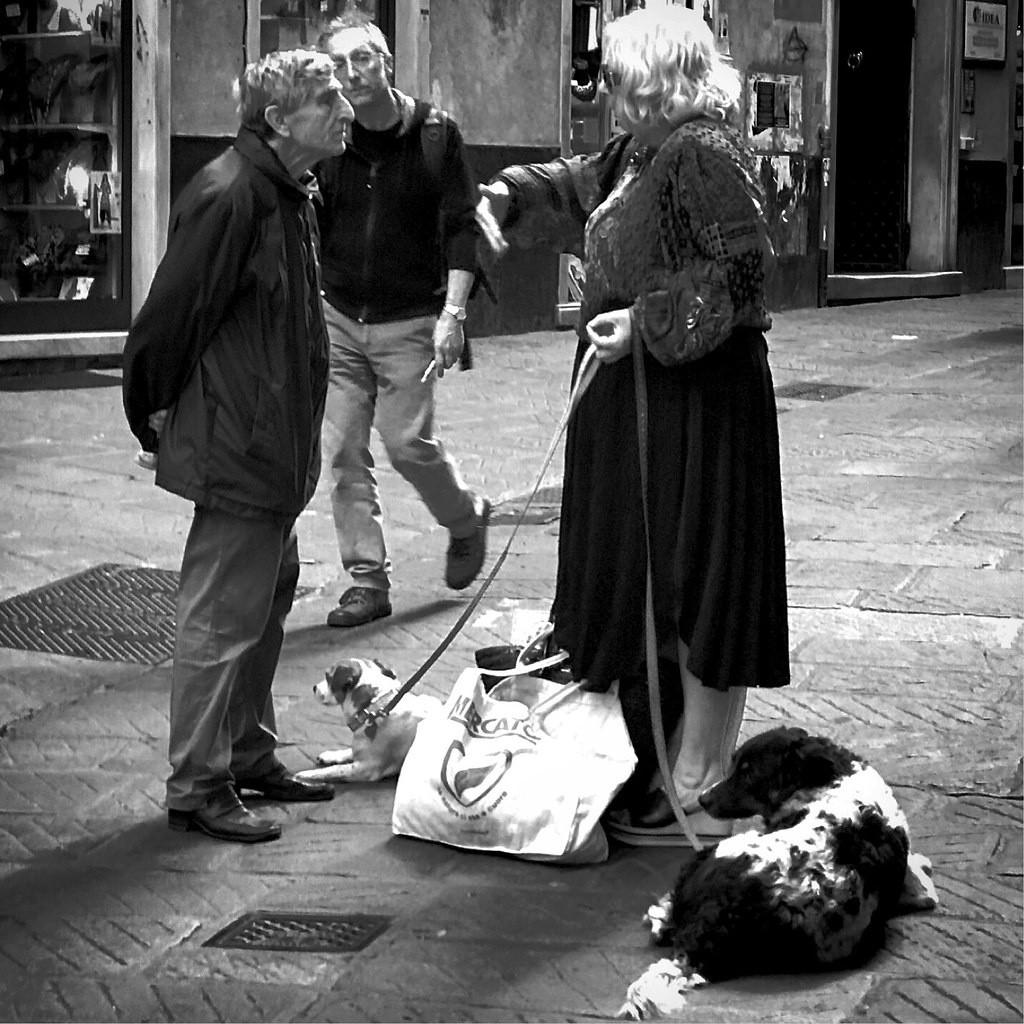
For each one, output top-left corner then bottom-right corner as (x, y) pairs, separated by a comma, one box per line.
(475, 6), (790, 839)
(309, 9), (491, 628)
(96, 174), (112, 228)
(122, 48), (356, 843)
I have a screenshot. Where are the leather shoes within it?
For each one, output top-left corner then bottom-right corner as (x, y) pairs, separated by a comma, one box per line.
(166, 781), (281, 844)
(233, 757), (336, 802)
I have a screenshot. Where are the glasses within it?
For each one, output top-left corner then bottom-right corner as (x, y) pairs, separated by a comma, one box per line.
(601, 62), (625, 92)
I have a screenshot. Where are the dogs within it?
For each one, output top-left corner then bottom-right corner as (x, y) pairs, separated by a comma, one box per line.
(289, 657), (446, 788)
(613, 724), (941, 1022)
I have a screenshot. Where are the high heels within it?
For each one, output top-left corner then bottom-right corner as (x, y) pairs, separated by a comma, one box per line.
(608, 780), (734, 846)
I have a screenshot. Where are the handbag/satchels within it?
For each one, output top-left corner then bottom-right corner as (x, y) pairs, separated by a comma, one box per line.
(391, 623), (638, 863)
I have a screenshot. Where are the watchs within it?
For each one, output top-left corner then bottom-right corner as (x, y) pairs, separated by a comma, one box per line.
(442, 303), (467, 321)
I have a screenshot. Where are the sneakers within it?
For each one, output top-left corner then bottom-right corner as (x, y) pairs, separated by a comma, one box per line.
(445, 499), (491, 590)
(327, 585), (392, 626)
